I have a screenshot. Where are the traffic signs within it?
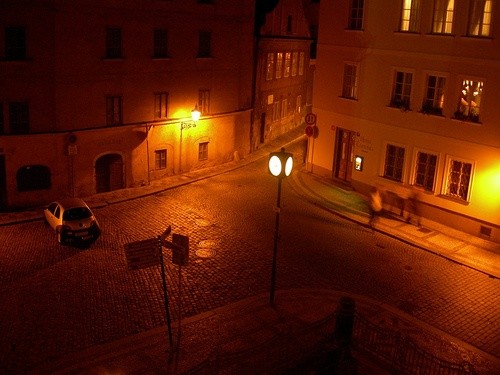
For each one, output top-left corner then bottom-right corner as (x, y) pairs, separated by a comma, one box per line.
(124, 225), (182, 271)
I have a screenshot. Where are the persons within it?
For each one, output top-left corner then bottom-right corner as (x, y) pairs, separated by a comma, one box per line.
(405, 187), (422, 226)
(368, 186), (383, 234)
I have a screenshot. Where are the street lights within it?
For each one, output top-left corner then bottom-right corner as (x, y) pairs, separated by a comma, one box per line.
(268, 147), (295, 308)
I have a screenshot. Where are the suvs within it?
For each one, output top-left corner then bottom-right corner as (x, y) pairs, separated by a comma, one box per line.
(42, 198), (100, 244)
